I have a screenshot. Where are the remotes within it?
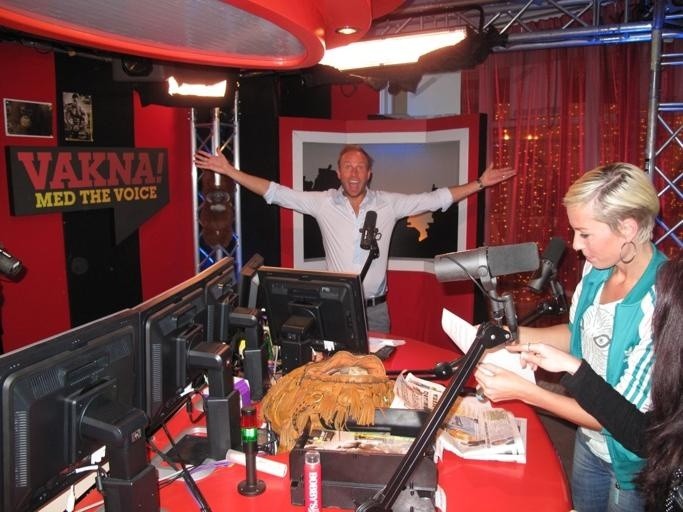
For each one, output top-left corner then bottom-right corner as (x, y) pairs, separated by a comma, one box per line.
(378, 344), (395, 361)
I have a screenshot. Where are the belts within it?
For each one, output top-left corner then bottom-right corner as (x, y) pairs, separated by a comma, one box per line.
(364, 295), (388, 306)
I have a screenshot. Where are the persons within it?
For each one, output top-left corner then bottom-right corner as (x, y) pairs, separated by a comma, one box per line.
(194, 143), (518, 333)
(474, 162), (672, 512)
(63, 94), (89, 140)
(505, 251), (683, 511)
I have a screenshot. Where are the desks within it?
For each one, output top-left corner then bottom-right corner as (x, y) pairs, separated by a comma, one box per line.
(69, 331), (575, 512)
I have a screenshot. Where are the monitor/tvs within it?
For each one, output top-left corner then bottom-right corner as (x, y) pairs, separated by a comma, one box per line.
(237, 252), (266, 317)
(197, 256), (267, 400)
(0, 306), (161, 512)
(132, 277), (243, 470)
(256, 266), (370, 377)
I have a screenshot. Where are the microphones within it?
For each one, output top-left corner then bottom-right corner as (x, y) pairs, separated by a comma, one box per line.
(433, 241), (539, 283)
(360, 210), (377, 250)
(528, 236), (566, 294)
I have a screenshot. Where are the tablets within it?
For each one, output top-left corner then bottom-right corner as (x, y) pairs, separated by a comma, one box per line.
(347, 408), (424, 434)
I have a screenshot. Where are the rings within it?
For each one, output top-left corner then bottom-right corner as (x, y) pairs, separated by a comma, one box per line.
(527, 342), (532, 352)
(477, 177), (485, 190)
